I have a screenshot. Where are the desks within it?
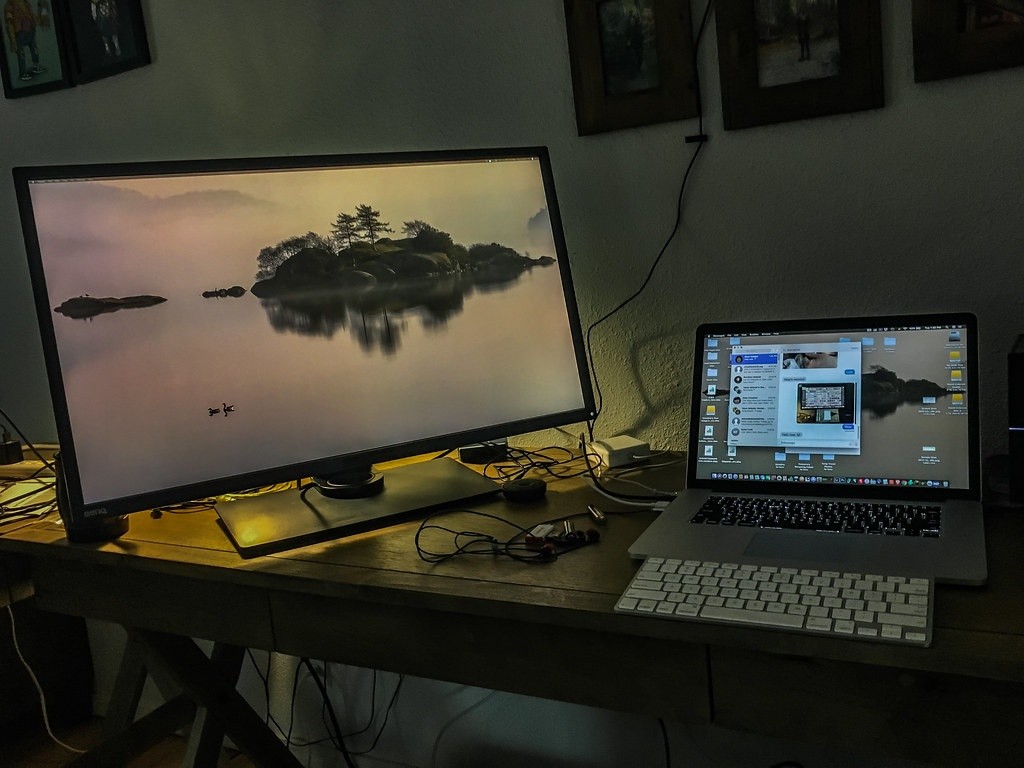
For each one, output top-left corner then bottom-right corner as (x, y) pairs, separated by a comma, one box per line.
(0, 445), (1024, 768)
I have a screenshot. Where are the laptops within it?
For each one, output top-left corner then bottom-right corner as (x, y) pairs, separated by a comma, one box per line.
(626, 310), (984, 588)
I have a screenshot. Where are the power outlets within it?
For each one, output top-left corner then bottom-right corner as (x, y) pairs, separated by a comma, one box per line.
(304, 659), (329, 687)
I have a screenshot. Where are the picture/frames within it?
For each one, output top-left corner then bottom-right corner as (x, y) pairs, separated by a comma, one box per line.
(0, 0), (69, 99)
(564, 0), (700, 138)
(714, 0), (886, 131)
(911, 0), (1024, 83)
(69, 0), (151, 87)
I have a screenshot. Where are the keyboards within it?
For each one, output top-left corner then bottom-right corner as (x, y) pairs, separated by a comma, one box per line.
(612, 555), (933, 647)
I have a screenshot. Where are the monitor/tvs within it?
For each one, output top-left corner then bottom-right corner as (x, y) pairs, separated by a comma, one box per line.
(12, 146), (597, 552)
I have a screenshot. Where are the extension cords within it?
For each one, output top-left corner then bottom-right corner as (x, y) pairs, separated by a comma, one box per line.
(0, 459), (57, 479)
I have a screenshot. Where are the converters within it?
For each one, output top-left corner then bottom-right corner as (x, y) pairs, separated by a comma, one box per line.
(459, 435), (509, 464)
